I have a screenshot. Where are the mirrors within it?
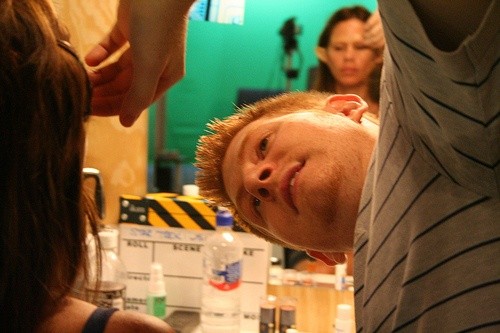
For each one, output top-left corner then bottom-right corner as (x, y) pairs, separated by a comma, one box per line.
(144, 0), (385, 278)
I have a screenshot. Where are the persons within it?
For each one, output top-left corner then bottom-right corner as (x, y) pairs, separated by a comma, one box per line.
(0, 0), (173, 333)
(85, 0), (500, 333)
(363, 10), (385, 48)
(316, 5), (383, 115)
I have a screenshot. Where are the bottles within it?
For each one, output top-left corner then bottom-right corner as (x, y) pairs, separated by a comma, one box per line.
(258, 305), (295, 333)
(81, 231), (127, 311)
(199, 210), (242, 333)
(146, 264), (166, 318)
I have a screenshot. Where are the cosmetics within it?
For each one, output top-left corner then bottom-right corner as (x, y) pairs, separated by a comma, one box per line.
(145, 263), (166, 320)
(279, 306), (295, 333)
(335, 304), (353, 333)
(259, 306), (275, 333)
